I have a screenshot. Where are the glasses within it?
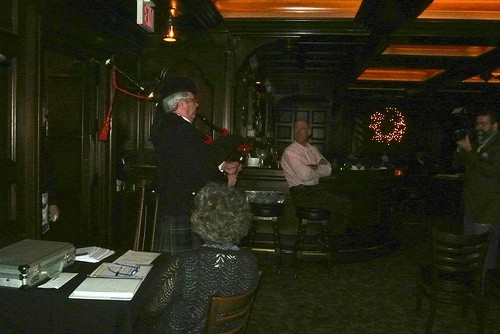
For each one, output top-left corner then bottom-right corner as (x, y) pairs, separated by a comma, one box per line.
(294, 127), (308, 132)
(186, 97), (201, 103)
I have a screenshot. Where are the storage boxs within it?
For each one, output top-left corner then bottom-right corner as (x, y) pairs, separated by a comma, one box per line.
(0, 239), (76, 289)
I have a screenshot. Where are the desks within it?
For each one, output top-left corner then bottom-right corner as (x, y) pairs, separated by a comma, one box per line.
(0, 249), (161, 334)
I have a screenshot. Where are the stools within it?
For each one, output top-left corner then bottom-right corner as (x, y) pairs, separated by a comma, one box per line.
(293, 206), (330, 267)
(248, 204), (283, 266)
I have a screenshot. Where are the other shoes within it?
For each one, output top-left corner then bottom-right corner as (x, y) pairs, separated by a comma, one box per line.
(322, 234), (353, 252)
(462, 268), (497, 285)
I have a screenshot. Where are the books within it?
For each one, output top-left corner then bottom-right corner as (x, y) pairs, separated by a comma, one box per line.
(68, 245), (161, 302)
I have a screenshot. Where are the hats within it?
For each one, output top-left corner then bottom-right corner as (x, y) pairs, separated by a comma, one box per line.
(161, 77), (197, 99)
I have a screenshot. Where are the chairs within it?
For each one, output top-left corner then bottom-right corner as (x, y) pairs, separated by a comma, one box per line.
(206, 271), (263, 334)
(415, 228), (493, 334)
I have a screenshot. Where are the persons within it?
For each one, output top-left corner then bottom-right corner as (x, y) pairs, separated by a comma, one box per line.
(141, 181), (259, 334)
(150, 77), (244, 252)
(457, 111), (500, 304)
(279, 118), (351, 248)
